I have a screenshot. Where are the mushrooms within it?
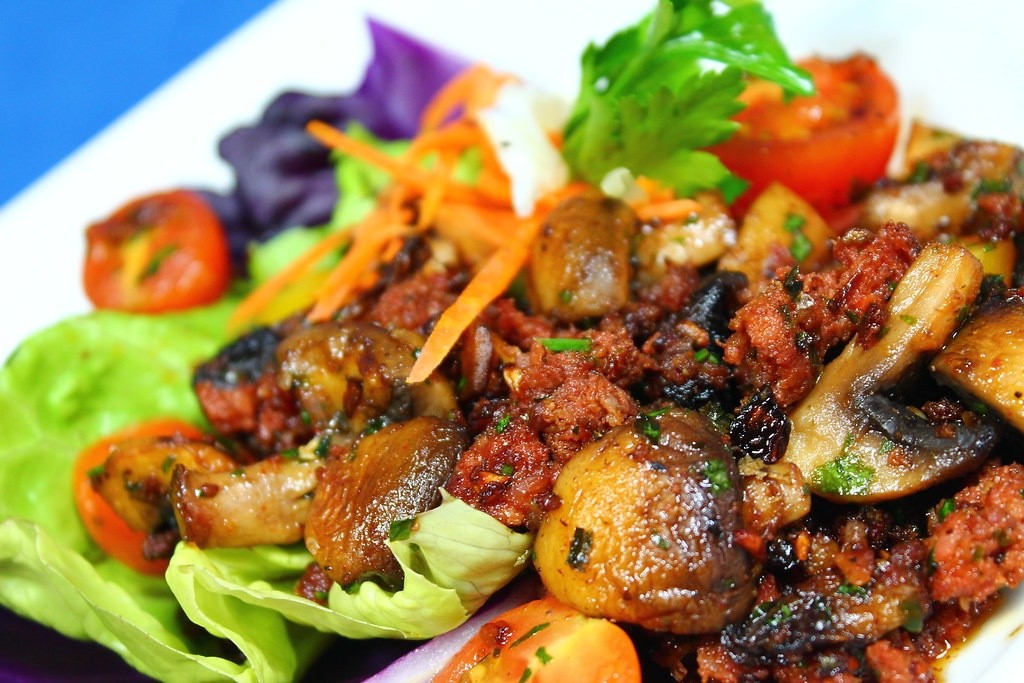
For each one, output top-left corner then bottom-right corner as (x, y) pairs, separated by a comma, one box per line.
(162, 184), (1024, 636)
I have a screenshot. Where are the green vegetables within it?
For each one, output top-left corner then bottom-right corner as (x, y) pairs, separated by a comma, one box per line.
(559, 0), (815, 203)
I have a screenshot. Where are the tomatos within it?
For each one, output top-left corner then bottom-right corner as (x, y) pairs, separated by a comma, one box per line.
(73, 419), (243, 576)
(81, 190), (225, 311)
(427, 593), (642, 683)
(710, 44), (905, 203)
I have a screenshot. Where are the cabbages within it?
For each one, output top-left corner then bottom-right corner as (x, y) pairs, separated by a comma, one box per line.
(0, 124), (540, 683)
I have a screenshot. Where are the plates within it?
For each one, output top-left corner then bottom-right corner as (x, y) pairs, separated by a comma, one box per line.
(0, 0), (1024, 683)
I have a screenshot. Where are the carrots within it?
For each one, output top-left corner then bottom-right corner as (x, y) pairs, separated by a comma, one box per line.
(226, 57), (585, 391)
